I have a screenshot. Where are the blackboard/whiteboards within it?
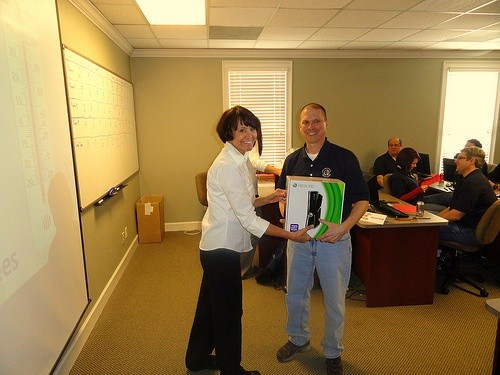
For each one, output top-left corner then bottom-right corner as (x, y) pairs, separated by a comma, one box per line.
(62, 44), (140, 213)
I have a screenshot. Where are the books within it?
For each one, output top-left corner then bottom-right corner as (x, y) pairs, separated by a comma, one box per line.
(356, 212), (388, 226)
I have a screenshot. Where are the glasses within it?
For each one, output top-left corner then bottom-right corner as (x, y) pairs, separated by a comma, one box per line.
(457, 156), (466, 160)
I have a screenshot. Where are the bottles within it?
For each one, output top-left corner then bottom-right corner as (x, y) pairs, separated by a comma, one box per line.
(439, 171), (444, 186)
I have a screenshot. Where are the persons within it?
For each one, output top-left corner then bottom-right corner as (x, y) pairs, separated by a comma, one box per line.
(185, 107), (315, 375)
(421, 147), (497, 263)
(391, 145), (453, 217)
(373, 137), (422, 193)
(272, 103), (369, 374)
(454, 139), (488, 183)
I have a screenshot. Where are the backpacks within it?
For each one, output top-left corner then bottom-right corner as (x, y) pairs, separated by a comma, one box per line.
(254, 241), (286, 284)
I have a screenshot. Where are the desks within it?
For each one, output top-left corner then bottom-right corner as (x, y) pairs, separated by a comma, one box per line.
(428, 181), (455, 193)
(350, 192), (449, 308)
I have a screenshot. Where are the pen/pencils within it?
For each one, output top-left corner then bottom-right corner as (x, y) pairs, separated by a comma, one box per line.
(444, 188), (448, 191)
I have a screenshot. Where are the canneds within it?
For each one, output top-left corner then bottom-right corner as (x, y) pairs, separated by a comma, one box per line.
(416, 201), (424, 217)
(439, 173), (444, 185)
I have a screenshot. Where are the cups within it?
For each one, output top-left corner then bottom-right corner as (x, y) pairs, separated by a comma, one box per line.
(416, 201), (425, 217)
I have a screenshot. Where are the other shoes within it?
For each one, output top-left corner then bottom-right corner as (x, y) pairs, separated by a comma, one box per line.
(241, 264), (253, 280)
(325, 355), (343, 375)
(186, 355), (220, 370)
(220, 365), (260, 375)
(276, 339), (312, 362)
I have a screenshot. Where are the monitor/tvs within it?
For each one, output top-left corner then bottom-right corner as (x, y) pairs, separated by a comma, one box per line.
(443, 159), (460, 192)
(414, 154), (431, 179)
(362, 171), (381, 209)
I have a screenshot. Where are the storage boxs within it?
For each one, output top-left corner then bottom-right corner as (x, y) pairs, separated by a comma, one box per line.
(285, 175), (345, 239)
(136, 195), (165, 244)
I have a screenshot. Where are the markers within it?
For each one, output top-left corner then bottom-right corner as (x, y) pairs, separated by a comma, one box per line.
(97, 199), (103, 205)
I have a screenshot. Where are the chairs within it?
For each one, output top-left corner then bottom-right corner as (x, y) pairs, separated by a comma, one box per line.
(196, 172), (208, 206)
(440, 199), (500, 297)
(389, 175), (423, 206)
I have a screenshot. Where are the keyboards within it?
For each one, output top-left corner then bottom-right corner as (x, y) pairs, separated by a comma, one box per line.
(379, 202), (409, 218)
